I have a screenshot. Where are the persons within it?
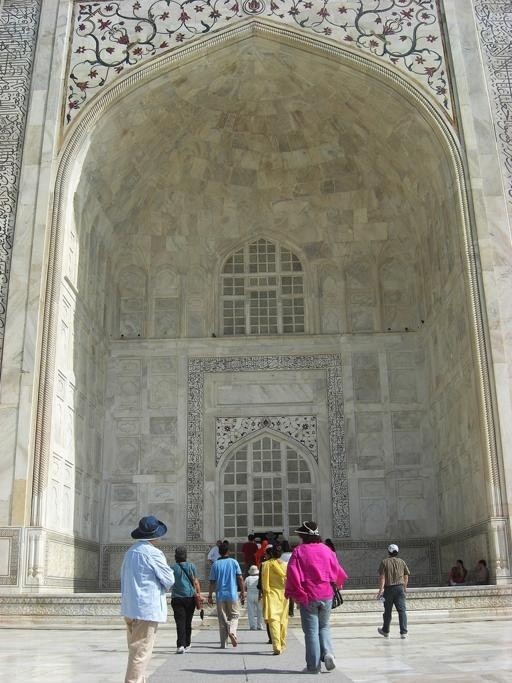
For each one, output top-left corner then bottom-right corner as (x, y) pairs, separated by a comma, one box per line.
(206, 531), (300, 655)
(120, 516), (175, 683)
(447, 559), (467, 586)
(168, 547), (204, 653)
(325, 538), (335, 552)
(375, 544), (410, 637)
(288, 520), (347, 672)
(474, 559), (489, 585)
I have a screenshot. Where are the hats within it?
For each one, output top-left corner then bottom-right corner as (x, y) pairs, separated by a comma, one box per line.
(293, 521), (319, 536)
(248, 565), (260, 575)
(174, 546), (187, 555)
(131, 516), (168, 540)
(388, 544), (398, 553)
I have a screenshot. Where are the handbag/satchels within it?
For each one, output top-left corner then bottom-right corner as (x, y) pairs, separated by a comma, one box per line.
(331, 585), (343, 609)
(196, 595), (202, 610)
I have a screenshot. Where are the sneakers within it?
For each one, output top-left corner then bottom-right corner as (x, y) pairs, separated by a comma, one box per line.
(250, 626), (264, 630)
(401, 634), (408, 639)
(229, 633), (238, 647)
(323, 654), (336, 671)
(302, 666), (321, 674)
(377, 627), (390, 637)
(177, 644), (192, 654)
